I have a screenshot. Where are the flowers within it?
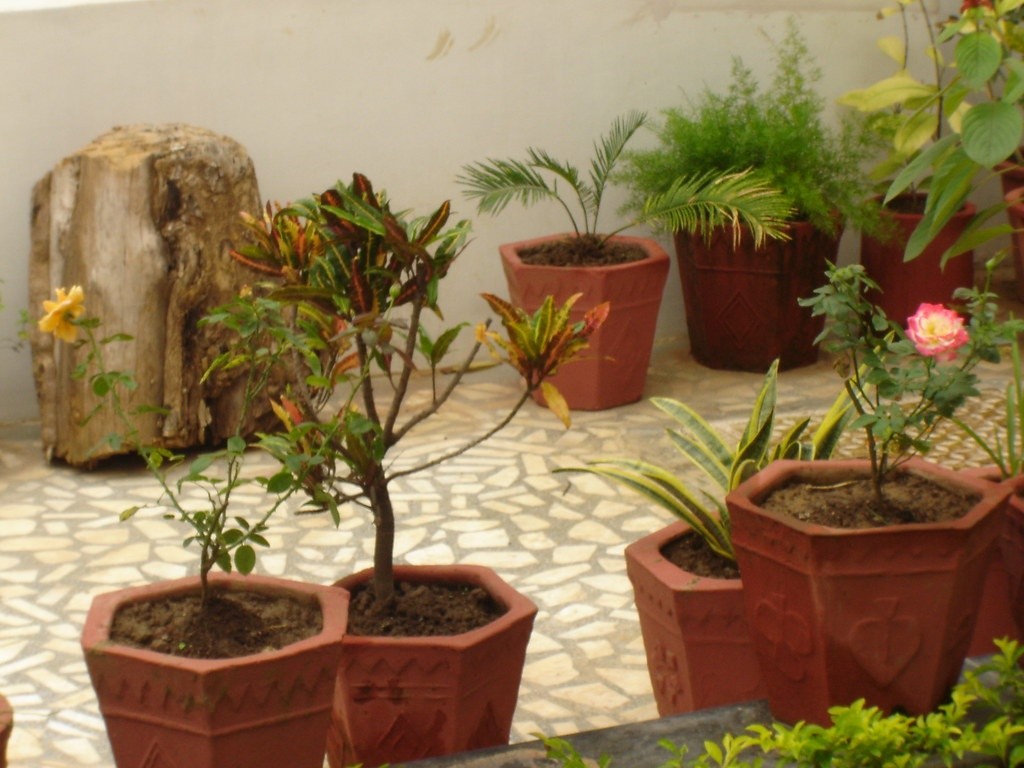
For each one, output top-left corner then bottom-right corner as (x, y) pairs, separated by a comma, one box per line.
(15, 250), (386, 613)
(793, 253), (1024, 504)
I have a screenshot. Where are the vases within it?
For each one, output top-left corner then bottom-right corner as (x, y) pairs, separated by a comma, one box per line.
(721, 458), (1014, 728)
(80, 572), (349, 767)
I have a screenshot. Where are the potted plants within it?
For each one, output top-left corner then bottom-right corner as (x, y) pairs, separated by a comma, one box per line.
(199, 3), (1022, 766)
